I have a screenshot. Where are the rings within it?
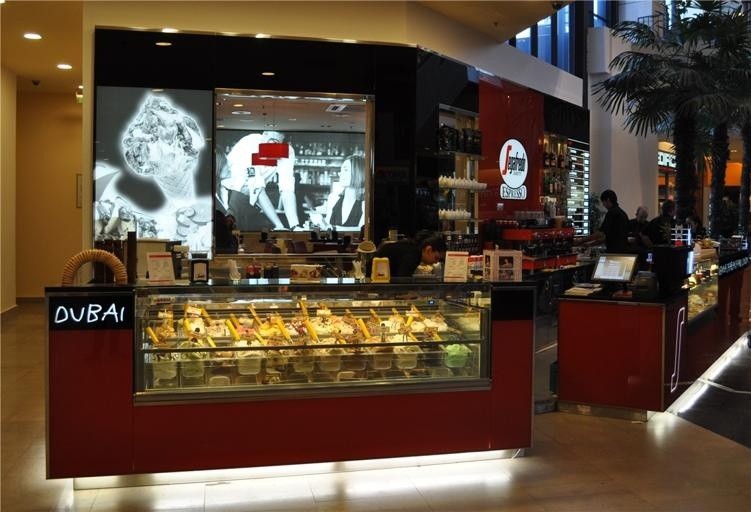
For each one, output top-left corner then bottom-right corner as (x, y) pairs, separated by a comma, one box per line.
(120, 93), (205, 207)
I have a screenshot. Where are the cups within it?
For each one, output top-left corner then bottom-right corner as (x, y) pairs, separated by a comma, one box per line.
(697, 272), (704, 283)
(388, 230), (398, 241)
(552, 217), (562, 228)
(648, 277), (658, 297)
(513, 210), (544, 226)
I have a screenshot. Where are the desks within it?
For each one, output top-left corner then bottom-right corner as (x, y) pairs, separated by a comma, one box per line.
(214, 250), (358, 278)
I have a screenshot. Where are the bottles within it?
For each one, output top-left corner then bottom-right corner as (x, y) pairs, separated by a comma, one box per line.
(331, 227), (337, 239)
(443, 232), (479, 254)
(245, 262), (279, 279)
(93, 238), (125, 283)
(325, 228), (331, 240)
(294, 158), (326, 166)
(299, 168), (331, 186)
(293, 140), (360, 157)
(541, 142), (572, 216)
(307, 206), (327, 230)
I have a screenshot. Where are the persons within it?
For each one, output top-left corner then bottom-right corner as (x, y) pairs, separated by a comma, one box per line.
(365, 230), (447, 276)
(684, 213), (706, 238)
(575, 190), (631, 254)
(630, 206), (649, 249)
(638, 199), (693, 247)
(214, 131), (365, 232)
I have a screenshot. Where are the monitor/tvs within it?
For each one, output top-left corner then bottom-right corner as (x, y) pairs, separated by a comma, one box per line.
(590, 253), (640, 282)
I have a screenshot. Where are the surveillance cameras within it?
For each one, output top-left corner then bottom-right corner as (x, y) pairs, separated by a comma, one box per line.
(551, 1), (564, 9)
(31, 80), (40, 86)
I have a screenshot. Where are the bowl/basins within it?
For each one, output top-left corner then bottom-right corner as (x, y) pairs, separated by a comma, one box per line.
(439, 208), (472, 219)
(437, 174), (487, 192)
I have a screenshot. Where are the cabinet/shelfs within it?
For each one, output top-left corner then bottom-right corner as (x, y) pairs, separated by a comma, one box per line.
(44, 282), (539, 491)
(412, 150), (485, 231)
(555, 255), (751, 421)
(294, 155), (346, 185)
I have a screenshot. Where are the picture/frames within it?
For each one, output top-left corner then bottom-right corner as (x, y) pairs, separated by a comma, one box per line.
(76, 173), (82, 208)
